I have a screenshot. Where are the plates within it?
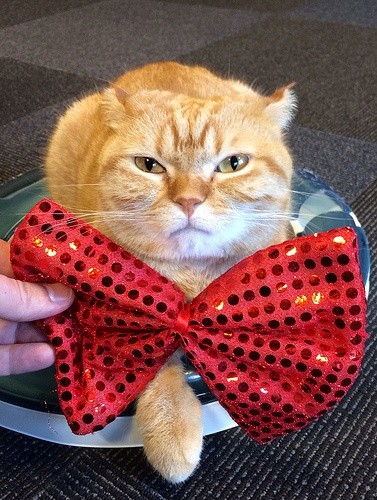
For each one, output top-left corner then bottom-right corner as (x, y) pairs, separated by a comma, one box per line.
(1, 165), (372, 448)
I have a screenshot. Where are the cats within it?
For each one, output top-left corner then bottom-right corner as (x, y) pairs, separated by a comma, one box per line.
(47, 60), (295, 484)
